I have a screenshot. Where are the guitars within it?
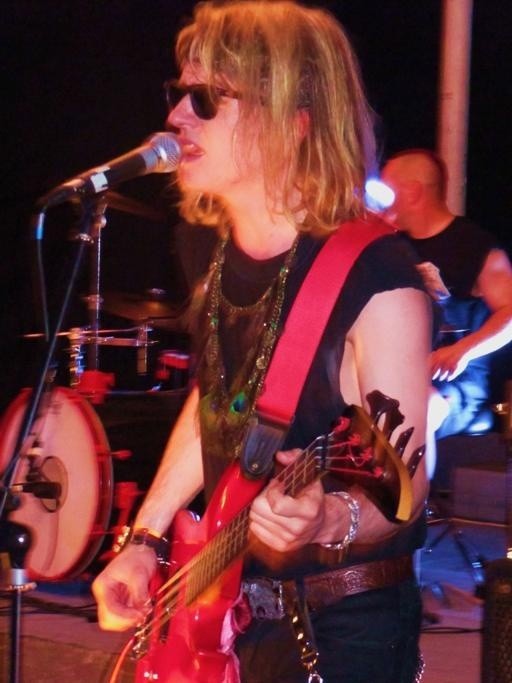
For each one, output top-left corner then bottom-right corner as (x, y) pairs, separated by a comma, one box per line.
(98, 390), (424, 680)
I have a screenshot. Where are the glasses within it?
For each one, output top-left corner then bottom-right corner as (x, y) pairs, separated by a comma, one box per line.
(164, 80), (312, 120)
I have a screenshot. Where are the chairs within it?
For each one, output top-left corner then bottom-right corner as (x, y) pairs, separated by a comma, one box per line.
(426, 354), (504, 599)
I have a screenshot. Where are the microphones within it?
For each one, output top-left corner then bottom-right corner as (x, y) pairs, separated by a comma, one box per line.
(35, 130), (183, 210)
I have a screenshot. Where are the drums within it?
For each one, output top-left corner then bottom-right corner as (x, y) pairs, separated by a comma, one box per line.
(1, 381), (188, 589)
(152, 352), (190, 382)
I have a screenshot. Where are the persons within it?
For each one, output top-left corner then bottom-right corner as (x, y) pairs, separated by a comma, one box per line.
(92, 0), (445, 683)
(379, 150), (512, 481)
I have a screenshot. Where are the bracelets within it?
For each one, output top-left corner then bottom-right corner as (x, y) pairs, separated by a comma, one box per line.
(320, 491), (360, 552)
(130, 528), (170, 558)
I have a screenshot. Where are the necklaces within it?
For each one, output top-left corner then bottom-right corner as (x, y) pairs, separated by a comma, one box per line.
(206, 238), (299, 459)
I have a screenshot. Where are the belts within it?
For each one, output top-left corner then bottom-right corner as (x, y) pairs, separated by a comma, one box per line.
(242, 554), (414, 621)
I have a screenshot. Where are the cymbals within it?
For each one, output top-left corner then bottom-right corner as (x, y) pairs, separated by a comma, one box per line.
(98, 284), (180, 330)
